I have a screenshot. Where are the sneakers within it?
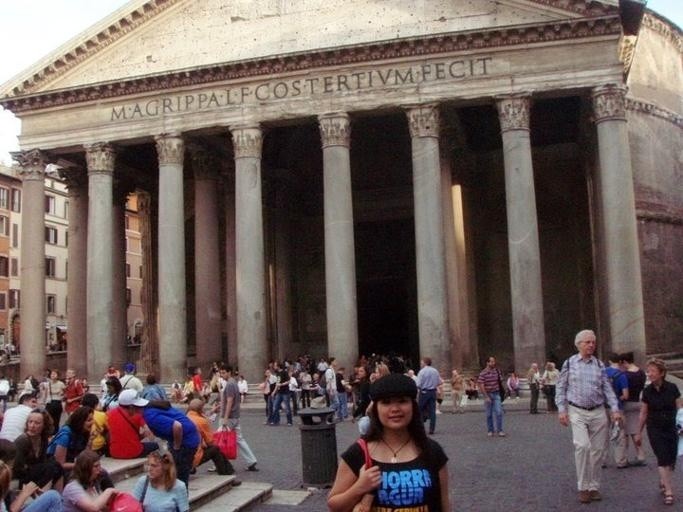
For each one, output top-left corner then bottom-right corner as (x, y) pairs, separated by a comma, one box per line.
(589, 490), (602, 500)
(599, 457), (649, 469)
(577, 490), (591, 504)
(435, 407), (466, 416)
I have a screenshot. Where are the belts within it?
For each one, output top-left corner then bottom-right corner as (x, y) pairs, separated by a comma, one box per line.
(568, 401), (602, 411)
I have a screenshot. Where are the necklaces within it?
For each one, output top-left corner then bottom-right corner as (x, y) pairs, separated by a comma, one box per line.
(380, 437), (411, 464)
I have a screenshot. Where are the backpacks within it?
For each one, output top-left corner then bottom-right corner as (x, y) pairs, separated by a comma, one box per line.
(74, 377), (89, 405)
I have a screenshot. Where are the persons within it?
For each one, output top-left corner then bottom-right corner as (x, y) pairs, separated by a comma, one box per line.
(450, 356), (518, 434)
(325, 375), (452, 512)
(637, 352), (673, 504)
(598, 353), (631, 469)
(262, 354), (444, 434)
(528, 362), (559, 414)
(554, 328), (620, 503)
(619, 351), (648, 467)
(2, 352), (258, 511)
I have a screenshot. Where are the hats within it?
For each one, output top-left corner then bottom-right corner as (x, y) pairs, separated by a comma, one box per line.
(368, 373), (417, 401)
(119, 388), (150, 407)
(124, 363), (134, 374)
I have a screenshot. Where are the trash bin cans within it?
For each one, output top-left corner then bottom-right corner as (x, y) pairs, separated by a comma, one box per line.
(297, 407), (338, 489)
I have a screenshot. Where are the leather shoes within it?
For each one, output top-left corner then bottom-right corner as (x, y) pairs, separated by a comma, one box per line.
(488, 431), (494, 436)
(496, 431), (506, 437)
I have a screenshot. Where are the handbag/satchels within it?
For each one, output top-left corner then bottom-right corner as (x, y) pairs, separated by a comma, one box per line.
(352, 439), (374, 512)
(107, 491), (144, 512)
(496, 369), (506, 402)
(212, 425), (236, 459)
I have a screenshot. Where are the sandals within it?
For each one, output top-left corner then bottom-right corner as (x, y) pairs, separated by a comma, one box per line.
(659, 481), (665, 495)
(663, 492), (673, 505)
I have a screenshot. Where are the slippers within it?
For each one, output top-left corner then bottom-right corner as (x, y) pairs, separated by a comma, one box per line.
(245, 466), (259, 472)
(208, 465), (217, 472)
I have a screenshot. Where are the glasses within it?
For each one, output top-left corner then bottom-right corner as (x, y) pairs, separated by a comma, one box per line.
(156, 448), (169, 464)
(94, 463), (100, 470)
